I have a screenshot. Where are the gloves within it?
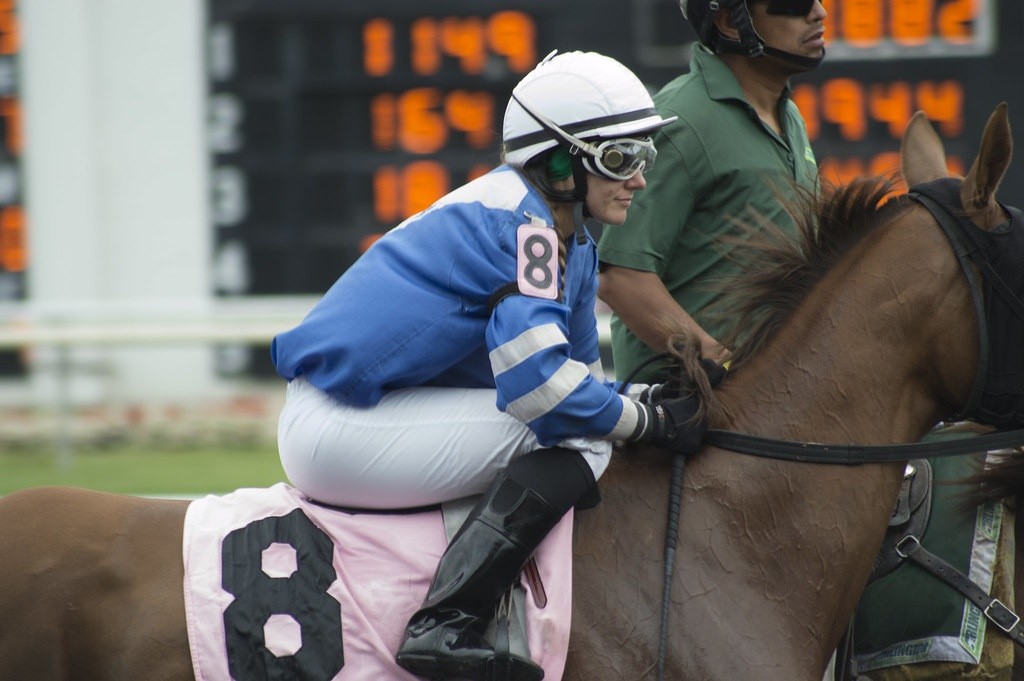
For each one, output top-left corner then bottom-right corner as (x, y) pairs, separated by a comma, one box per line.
(640, 359), (729, 403)
(637, 386), (712, 457)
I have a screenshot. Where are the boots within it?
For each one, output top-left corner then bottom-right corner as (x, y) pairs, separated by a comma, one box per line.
(392, 462), (549, 681)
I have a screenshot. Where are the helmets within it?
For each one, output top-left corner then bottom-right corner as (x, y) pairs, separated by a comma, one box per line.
(676, 0), (825, 33)
(497, 48), (681, 167)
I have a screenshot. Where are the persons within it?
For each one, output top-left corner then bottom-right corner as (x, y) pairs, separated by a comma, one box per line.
(589, 0), (829, 389)
(267, 44), (728, 681)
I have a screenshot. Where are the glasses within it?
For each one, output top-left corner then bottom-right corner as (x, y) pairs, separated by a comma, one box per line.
(758, 0), (826, 17)
(581, 140), (659, 181)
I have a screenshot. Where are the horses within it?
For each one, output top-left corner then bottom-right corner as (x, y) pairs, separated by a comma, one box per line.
(0, 101), (1024, 681)
